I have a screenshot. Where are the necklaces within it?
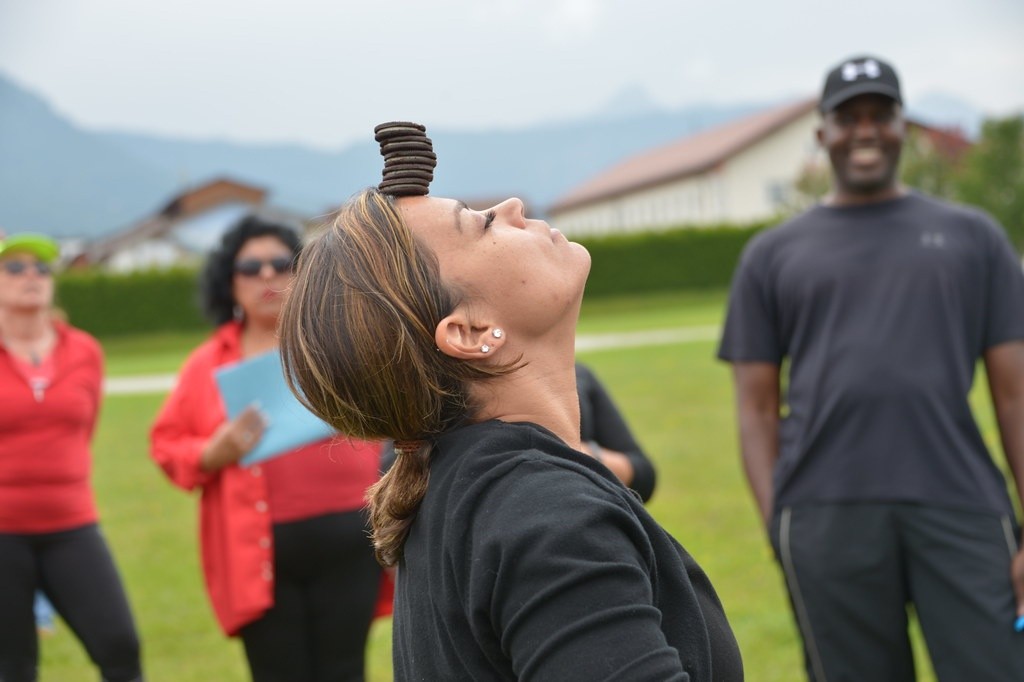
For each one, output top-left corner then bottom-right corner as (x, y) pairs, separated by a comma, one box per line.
(29, 350), (41, 367)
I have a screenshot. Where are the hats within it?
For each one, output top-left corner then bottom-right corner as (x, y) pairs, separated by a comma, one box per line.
(818, 56), (903, 111)
(0, 233), (63, 267)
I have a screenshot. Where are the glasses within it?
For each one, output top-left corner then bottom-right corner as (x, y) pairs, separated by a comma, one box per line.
(5, 260), (50, 276)
(232, 258), (294, 277)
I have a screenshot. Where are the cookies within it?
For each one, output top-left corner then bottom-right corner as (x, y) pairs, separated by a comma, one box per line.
(374, 121), (437, 196)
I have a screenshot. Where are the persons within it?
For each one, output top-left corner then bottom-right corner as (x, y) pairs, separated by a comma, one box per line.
(0, 232), (143, 682)
(719, 56), (1024, 682)
(278, 186), (745, 682)
(380, 359), (656, 503)
(151, 218), (399, 682)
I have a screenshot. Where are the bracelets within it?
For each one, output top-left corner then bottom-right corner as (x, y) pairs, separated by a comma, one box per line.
(588, 440), (600, 462)
